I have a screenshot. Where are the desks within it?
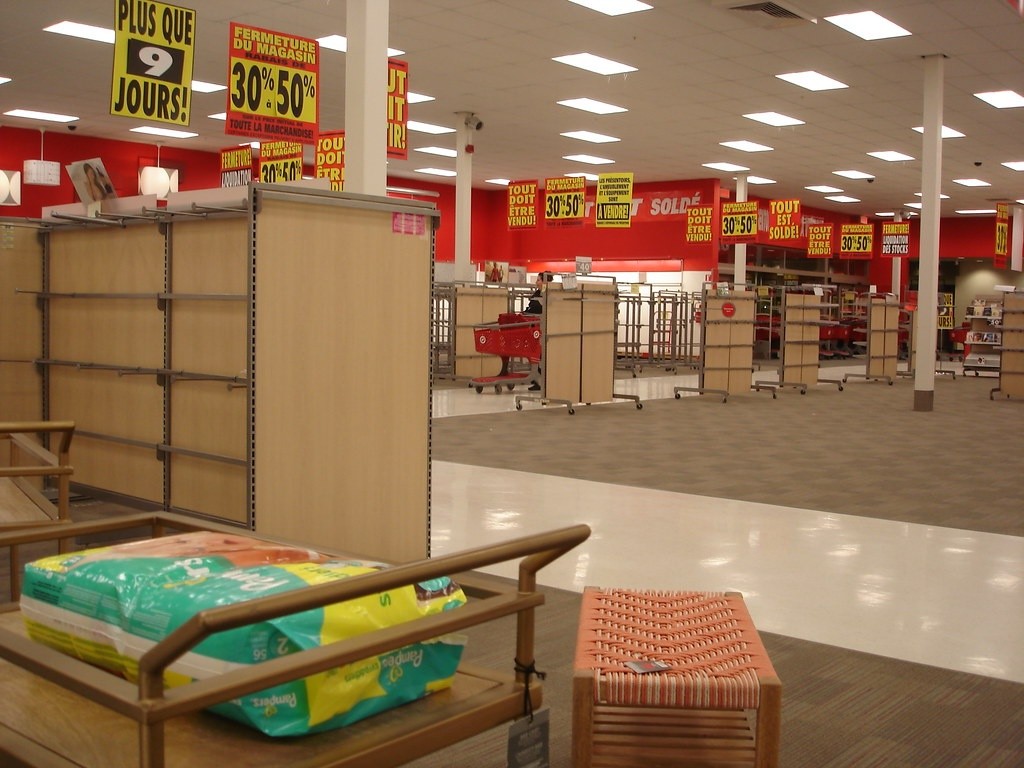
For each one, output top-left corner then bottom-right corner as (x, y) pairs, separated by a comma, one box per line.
(0, 416), (76, 529)
(0, 512), (587, 768)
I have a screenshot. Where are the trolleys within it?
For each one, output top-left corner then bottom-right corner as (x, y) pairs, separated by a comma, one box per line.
(467, 309), (544, 396)
(946, 322), (972, 364)
(0, 506), (592, 768)
(757, 303), (942, 361)
(0, 419), (77, 610)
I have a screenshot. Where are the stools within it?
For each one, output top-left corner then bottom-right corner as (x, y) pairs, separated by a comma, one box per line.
(571, 585), (780, 768)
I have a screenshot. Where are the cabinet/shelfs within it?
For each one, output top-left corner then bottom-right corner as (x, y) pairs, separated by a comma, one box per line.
(961, 316), (1002, 376)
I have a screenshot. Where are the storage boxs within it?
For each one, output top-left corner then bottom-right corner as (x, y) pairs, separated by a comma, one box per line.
(970, 344), (993, 353)
(971, 318), (987, 332)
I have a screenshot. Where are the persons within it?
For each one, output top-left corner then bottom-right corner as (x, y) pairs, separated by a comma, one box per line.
(994, 320), (1001, 325)
(490, 262), (499, 282)
(978, 358), (984, 365)
(520, 270), (554, 391)
(974, 333), (983, 342)
(110, 530), (265, 558)
(83, 163), (114, 202)
(498, 265), (504, 283)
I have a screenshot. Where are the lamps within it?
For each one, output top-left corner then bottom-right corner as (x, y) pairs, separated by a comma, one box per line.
(138, 142), (179, 199)
(24, 125), (61, 186)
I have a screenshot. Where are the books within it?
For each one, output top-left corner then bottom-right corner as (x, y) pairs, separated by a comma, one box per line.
(967, 304), (1001, 317)
(966, 331), (1001, 343)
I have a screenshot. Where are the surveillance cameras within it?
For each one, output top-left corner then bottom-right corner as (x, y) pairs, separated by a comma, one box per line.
(867, 179), (874, 183)
(975, 162), (981, 166)
(68, 126), (76, 130)
(468, 117), (483, 130)
